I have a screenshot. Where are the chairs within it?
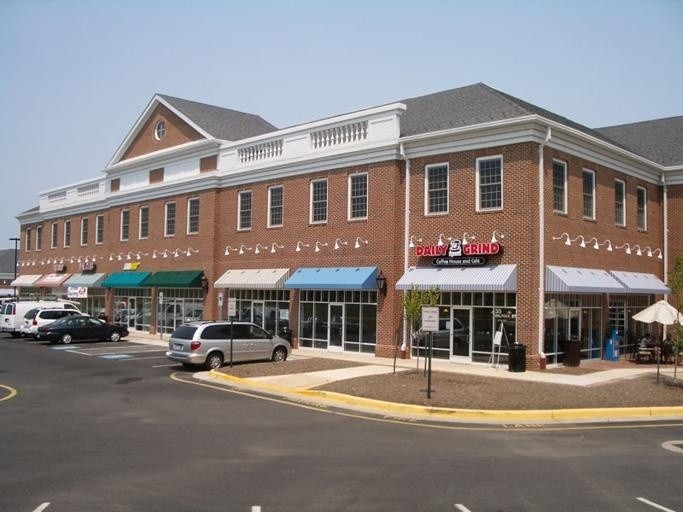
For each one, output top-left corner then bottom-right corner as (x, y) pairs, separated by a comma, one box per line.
(624, 332), (679, 363)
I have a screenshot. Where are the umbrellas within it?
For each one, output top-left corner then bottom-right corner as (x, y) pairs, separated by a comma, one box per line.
(631, 300), (683, 383)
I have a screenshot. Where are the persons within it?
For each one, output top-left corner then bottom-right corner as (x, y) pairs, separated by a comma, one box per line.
(640, 333), (656, 361)
(663, 333), (674, 355)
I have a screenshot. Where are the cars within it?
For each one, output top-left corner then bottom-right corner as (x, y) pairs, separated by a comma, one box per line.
(322, 317), (368, 335)
(39, 314), (129, 344)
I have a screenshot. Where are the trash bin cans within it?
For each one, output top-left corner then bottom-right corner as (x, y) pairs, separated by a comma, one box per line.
(566, 340), (581, 366)
(97, 312), (105, 319)
(509, 343), (526, 371)
(279, 332), (291, 345)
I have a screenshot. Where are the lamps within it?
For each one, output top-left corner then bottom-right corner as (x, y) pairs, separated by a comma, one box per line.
(408, 235), (423, 248)
(354, 237), (369, 249)
(270, 242), (284, 254)
(109, 250), (149, 261)
(200, 274), (209, 289)
(551, 233), (664, 260)
(17, 259), (39, 266)
(377, 270), (386, 292)
(490, 231), (502, 243)
(70, 254), (104, 264)
(238, 244), (252, 255)
(437, 233), (452, 246)
(334, 238), (348, 250)
(254, 243), (268, 254)
(41, 256), (69, 266)
(461, 232), (476, 246)
(151, 246), (200, 259)
(314, 241), (328, 253)
(295, 241), (310, 251)
(224, 245), (238, 256)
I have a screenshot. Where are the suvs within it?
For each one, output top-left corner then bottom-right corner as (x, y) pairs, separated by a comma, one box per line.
(21, 306), (106, 340)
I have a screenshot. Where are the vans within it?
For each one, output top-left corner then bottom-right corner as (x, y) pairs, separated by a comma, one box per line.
(157, 302), (202, 320)
(0, 299), (82, 336)
(166, 320), (292, 370)
(413, 316), (467, 348)
